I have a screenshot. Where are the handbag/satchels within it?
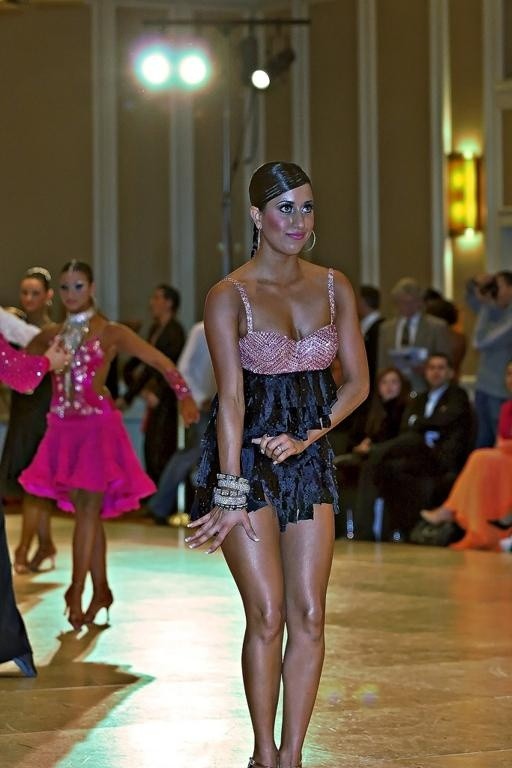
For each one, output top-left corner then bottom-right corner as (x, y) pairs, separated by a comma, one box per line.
(409, 520), (453, 547)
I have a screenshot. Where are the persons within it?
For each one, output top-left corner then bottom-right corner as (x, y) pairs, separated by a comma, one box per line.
(0, 308), (43, 678)
(2, 334), (69, 395)
(1, 266), (64, 572)
(182, 160), (369, 767)
(17, 259), (201, 633)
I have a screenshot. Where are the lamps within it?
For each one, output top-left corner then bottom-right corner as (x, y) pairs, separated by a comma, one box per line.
(128, 16), (313, 99)
(443, 146), (486, 237)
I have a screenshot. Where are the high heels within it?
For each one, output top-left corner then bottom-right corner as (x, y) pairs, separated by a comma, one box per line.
(420, 507), (452, 525)
(28, 541), (56, 570)
(64, 589), (84, 630)
(13, 542), (29, 573)
(84, 589), (113, 624)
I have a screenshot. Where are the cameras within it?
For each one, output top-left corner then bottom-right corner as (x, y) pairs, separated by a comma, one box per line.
(480, 280), (498, 299)
